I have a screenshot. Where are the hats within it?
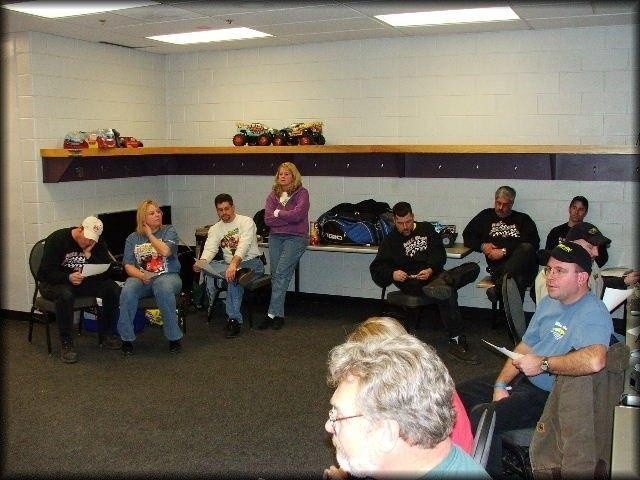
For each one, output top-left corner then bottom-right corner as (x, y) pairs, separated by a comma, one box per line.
(82, 216), (103, 243)
(536, 242), (592, 271)
(566, 222), (611, 246)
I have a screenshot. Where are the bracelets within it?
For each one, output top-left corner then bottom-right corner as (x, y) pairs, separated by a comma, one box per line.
(494, 383), (507, 387)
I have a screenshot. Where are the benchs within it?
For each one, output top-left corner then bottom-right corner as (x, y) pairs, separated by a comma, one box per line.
(475, 268), (633, 329)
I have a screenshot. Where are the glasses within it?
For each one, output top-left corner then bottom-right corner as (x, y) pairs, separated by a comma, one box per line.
(328, 411), (363, 428)
(545, 266), (568, 275)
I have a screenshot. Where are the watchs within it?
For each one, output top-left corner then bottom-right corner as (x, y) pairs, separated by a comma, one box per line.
(540, 357), (552, 376)
(501, 247), (506, 256)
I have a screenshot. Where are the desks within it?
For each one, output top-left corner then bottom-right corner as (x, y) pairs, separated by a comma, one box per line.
(258, 243), (474, 293)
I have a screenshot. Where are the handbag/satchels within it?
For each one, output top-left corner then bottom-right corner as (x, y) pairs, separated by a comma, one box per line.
(318, 199), (396, 246)
(254, 209), (269, 235)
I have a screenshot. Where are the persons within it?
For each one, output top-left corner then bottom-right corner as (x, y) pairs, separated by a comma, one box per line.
(321, 315), (476, 479)
(375, 200), (483, 366)
(38, 215), (123, 365)
(533, 220), (640, 309)
(192, 193), (266, 339)
(544, 195), (610, 269)
(324, 334), (494, 480)
(455, 240), (615, 479)
(461, 184), (541, 342)
(256, 160), (310, 330)
(115, 198), (184, 356)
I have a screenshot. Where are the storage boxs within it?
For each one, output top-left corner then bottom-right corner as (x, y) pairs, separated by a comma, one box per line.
(98, 205), (196, 284)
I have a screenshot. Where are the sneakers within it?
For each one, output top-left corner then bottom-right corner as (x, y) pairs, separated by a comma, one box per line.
(102, 333), (122, 349)
(238, 268), (255, 284)
(169, 340), (182, 353)
(61, 336), (78, 362)
(120, 340), (133, 354)
(226, 319), (240, 337)
(273, 317), (283, 329)
(486, 286), (500, 302)
(254, 317), (271, 329)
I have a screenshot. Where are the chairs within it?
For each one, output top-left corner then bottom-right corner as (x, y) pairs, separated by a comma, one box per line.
(28, 238), (104, 359)
(195, 233), (271, 332)
(471, 341), (630, 480)
(471, 401), (497, 469)
(379, 243), (447, 337)
(137, 294), (187, 336)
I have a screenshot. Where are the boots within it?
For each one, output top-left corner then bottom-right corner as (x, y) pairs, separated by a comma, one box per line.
(423, 272), (453, 300)
(449, 335), (480, 365)
